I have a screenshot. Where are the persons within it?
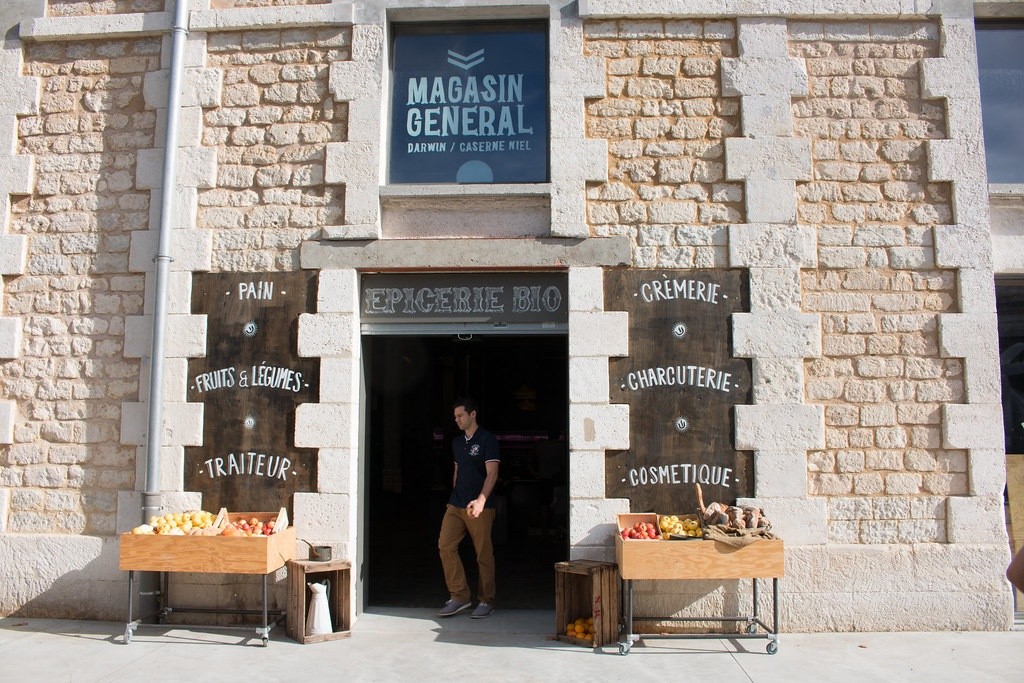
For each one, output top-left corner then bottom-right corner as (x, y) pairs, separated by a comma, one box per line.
(437, 400), (503, 618)
(525, 431), (559, 539)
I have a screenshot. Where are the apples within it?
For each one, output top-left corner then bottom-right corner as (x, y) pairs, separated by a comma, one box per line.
(622, 516), (702, 540)
(567, 617), (593, 640)
(132, 510), (277, 537)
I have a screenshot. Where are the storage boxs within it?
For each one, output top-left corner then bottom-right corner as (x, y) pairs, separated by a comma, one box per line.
(217, 506), (290, 534)
(285, 559), (351, 646)
(658, 514), (702, 539)
(614, 513), (662, 540)
(545, 559), (627, 648)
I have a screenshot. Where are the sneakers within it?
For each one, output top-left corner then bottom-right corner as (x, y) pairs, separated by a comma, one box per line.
(470, 603), (495, 618)
(436, 599), (472, 615)
